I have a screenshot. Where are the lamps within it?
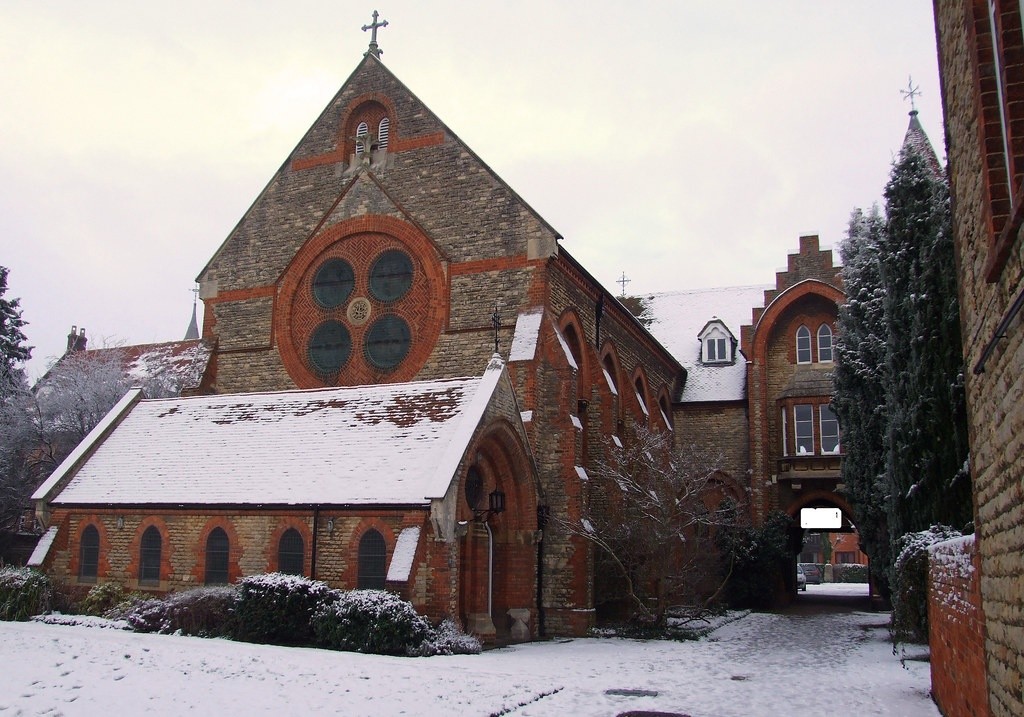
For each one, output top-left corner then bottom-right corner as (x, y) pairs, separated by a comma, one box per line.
(472, 482), (506, 522)
